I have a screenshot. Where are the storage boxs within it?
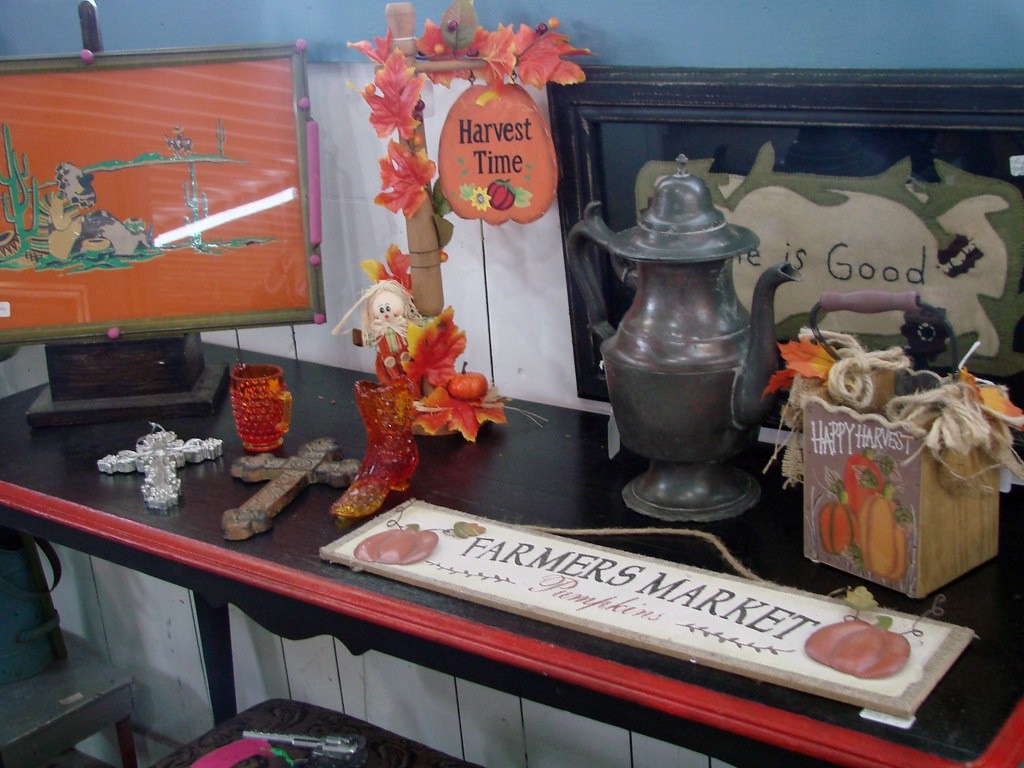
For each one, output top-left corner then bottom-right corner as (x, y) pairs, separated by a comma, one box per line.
(798, 365), (1005, 599)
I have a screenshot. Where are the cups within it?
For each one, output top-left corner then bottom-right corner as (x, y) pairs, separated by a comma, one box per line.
(231, 365), (290, 449)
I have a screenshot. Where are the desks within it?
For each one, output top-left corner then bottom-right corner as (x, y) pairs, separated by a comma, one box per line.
(0, 335), (1024, 768)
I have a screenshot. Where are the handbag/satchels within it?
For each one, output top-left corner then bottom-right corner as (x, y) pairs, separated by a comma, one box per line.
(0, 533), (68, 685)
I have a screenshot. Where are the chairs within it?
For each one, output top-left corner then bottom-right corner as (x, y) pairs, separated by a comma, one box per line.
(0, 628), (138, 768)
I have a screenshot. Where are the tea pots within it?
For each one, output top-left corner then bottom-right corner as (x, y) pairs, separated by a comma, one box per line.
(566, 153), (802, 522)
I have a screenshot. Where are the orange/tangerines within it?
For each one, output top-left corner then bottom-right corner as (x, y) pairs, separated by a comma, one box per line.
(447, 361), (487, 400)
